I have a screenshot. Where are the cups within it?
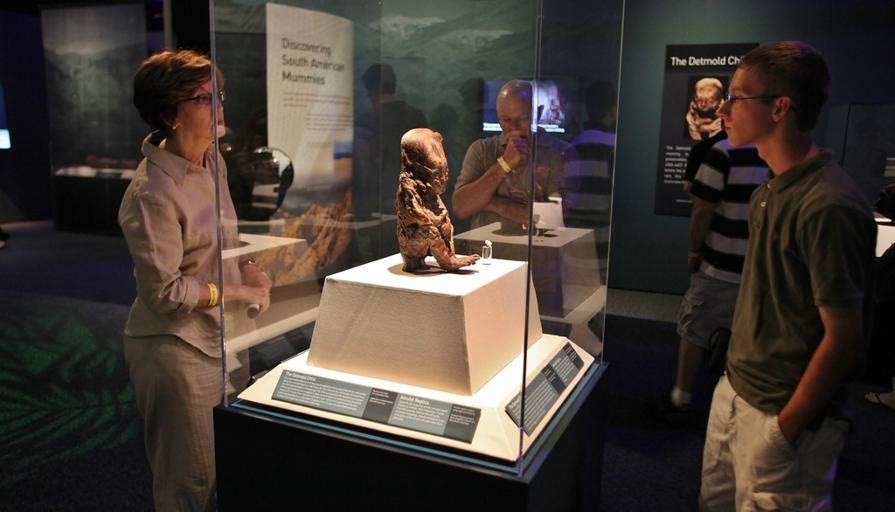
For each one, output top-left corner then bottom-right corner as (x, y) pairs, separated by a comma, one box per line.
(480, 247), (492, 266)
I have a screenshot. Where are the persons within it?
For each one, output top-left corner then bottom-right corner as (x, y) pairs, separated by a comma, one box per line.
(697, 41), (879, 512)
(397, 127), (479, 269)
(560, 76), (624, 286)
(351, 62), (428, 211)
(643, 134), (836, 424)
(686, 78), (726, 141)
(450, 79), (566, 231)
(117, 49), (272, 512)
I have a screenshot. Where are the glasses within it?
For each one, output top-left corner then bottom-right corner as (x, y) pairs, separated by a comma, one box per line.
(171, 88), (227, 107)
(726, 92), (777, 105)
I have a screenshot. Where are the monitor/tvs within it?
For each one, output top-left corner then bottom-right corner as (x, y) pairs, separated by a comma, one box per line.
(480, 72), (578, 143)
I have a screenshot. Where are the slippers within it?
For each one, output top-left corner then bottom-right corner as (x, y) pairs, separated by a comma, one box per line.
(861, 389), (895, 412)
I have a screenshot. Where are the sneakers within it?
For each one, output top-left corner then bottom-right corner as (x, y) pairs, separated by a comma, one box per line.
(644, 393), (695, 426)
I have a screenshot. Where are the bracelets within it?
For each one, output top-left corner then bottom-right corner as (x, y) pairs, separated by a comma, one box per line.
(686, 249), (702, 261)
(497, 156), (512, 174)
(208, 281), (219, 307)
(238, 259), (257, 267)
